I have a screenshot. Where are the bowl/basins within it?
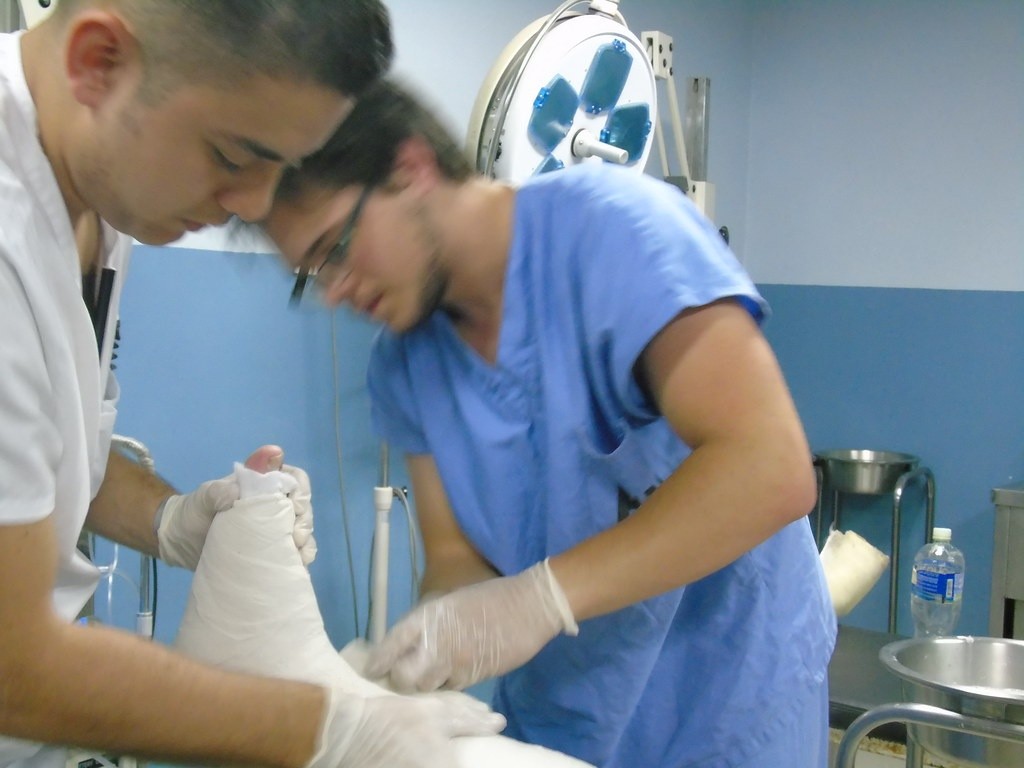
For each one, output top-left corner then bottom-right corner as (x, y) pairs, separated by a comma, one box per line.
(814, 448), (918, 496)
(878, 636), (1024, 768)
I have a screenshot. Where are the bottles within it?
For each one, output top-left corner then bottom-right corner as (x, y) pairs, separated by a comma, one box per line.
(911, 528), (964, 639)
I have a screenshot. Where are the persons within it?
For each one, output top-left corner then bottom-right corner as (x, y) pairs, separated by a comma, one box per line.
(260, 80), (839, 768)
(0, 0), (507, 767)
(178, 446), (596, 768)
